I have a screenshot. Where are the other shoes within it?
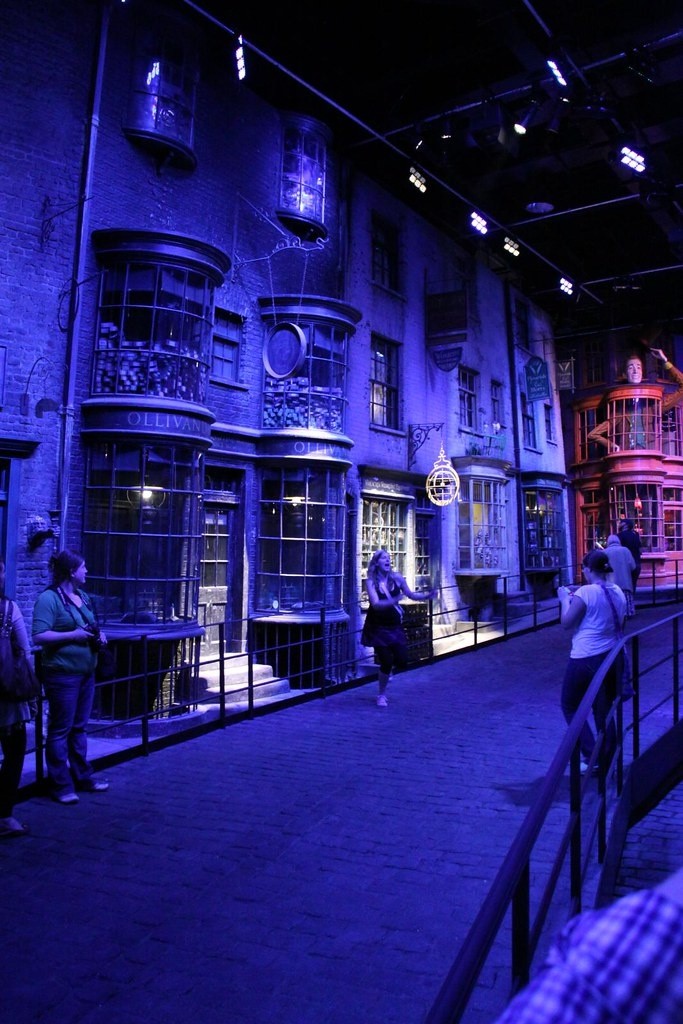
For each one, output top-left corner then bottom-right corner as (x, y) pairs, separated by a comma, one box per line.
(1, 816), (30, 838)
(376, 695), (389, 707)
(75, 777), (113, 793)
(563, 761), (599, 776)
(46, 789), (81, 806)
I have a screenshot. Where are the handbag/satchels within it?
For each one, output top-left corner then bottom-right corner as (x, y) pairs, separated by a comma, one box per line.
(0, 597), (42, 702)
(620, 649), (635, 702)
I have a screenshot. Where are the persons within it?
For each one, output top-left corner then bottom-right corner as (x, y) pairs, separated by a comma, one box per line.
(585, 348), (683, 549)
(557, 550), (628, 776)
(361, 549), (441, 707)
(605, 520), (643, 619)
(0, 562), (36, 836)
(31, 549), (108, 803)
